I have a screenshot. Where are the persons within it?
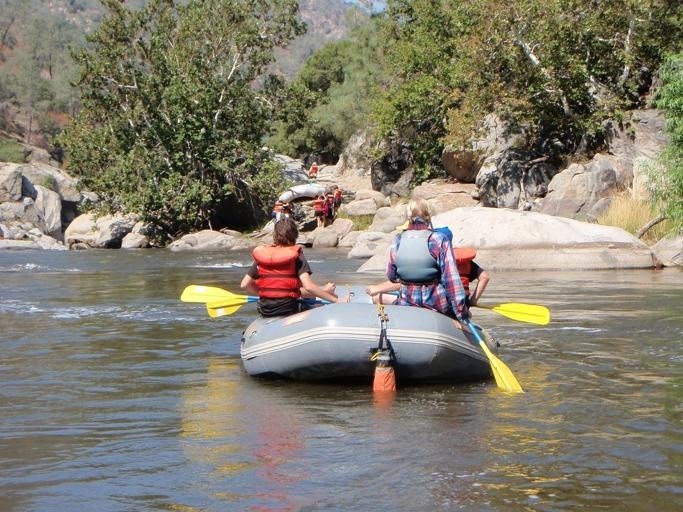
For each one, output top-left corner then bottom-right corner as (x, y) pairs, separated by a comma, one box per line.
(272, 162), (342, 230)
(240, 219), (349, 317)
(300, 282), (336, 298)
(387, 196), (472, 321)
(364, 227), (490, 309)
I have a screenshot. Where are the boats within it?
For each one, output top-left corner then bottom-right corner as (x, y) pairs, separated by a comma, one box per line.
(271, 184), (332, 229)
(238, 283), (500, 384)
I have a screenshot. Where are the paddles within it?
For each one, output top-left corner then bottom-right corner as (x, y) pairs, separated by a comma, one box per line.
(181, 284), (331, 304)
(468, 318), (522, 393)
(207, 297), (330, 317)
(471, 302), (550, 325)
